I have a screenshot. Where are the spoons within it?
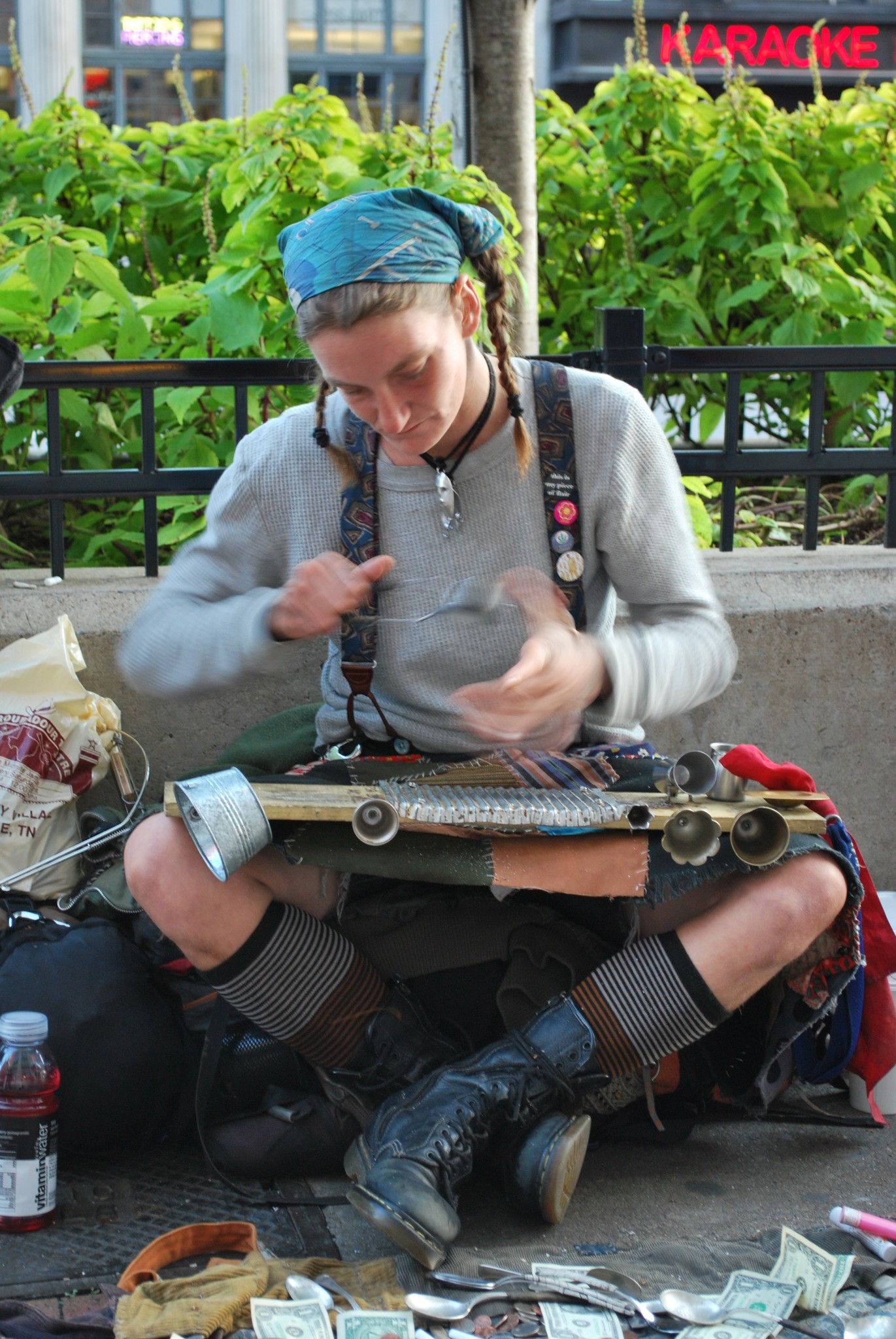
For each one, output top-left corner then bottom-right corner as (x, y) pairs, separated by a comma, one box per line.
(404, 1291), (570, 1322)
(568, 1269), (641, 1298)
(285, 1275), (344, 1314)
(430, 1272), (632, 1317)
(826, 1305), (896, 1339)
(536, 1270), (689, 1333)
(659, 1288), (842, 1339)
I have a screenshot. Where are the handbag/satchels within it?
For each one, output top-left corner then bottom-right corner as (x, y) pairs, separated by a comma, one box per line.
(0, 613), (119, 898)
(0, 916), (197, 1155)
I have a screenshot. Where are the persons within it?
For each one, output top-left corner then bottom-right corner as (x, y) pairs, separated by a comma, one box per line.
(115, 186), (865, 1275)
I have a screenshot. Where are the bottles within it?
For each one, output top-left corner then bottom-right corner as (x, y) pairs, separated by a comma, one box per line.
(0, 1011), (62, 1232)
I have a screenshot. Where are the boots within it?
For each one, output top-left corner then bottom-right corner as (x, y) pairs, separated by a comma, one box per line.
(316, 997), (593, 1226)
(342, 984), (610, 1270)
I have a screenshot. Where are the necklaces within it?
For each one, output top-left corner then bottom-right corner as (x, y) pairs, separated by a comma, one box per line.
(419, 350), (496, 539)
